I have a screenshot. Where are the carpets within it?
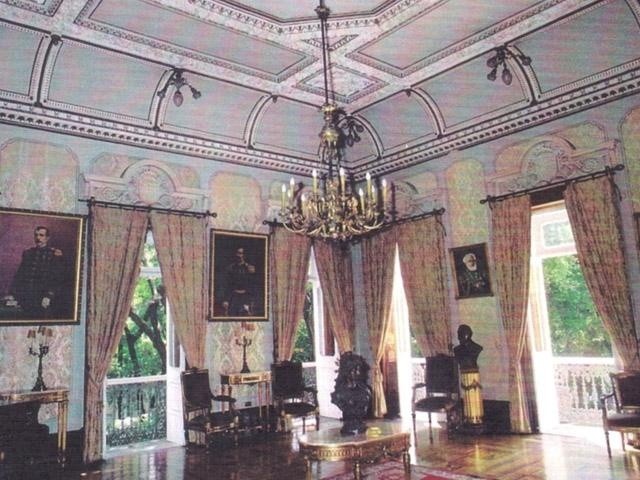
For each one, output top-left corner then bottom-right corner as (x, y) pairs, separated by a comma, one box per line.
(317, 459), (487, 480)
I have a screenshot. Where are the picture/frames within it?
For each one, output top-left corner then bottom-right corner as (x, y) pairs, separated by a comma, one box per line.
(448, 242), (494, 300)
(0, 207), (86, 326)
(209, 228), (270, 321)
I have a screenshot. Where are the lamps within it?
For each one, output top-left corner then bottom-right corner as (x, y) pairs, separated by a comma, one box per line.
(487, 46), (531, 85)
(279, 0), (390, 242)
(156, 67), (201, 107)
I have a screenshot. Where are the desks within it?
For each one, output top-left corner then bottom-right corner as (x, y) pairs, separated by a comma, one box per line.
(299, 423), (410, 480)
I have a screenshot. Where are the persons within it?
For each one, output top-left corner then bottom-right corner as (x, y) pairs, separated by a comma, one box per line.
(220, 244), (260, 316)
(1, 224), (67, 317)
(451, 323), (484, 370)
(458, 251), (491, 298)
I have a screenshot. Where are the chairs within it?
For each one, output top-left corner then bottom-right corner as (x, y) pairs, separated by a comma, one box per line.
(180, 368), (239, 454)
(412, 354), (458, 432)
(270, 360), (320, 434)
(600, 372), (640, 458)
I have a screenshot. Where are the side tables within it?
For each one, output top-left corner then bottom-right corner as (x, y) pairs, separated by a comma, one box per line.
(222, 370), (272, 418)
(0, 387), (68, 471)
